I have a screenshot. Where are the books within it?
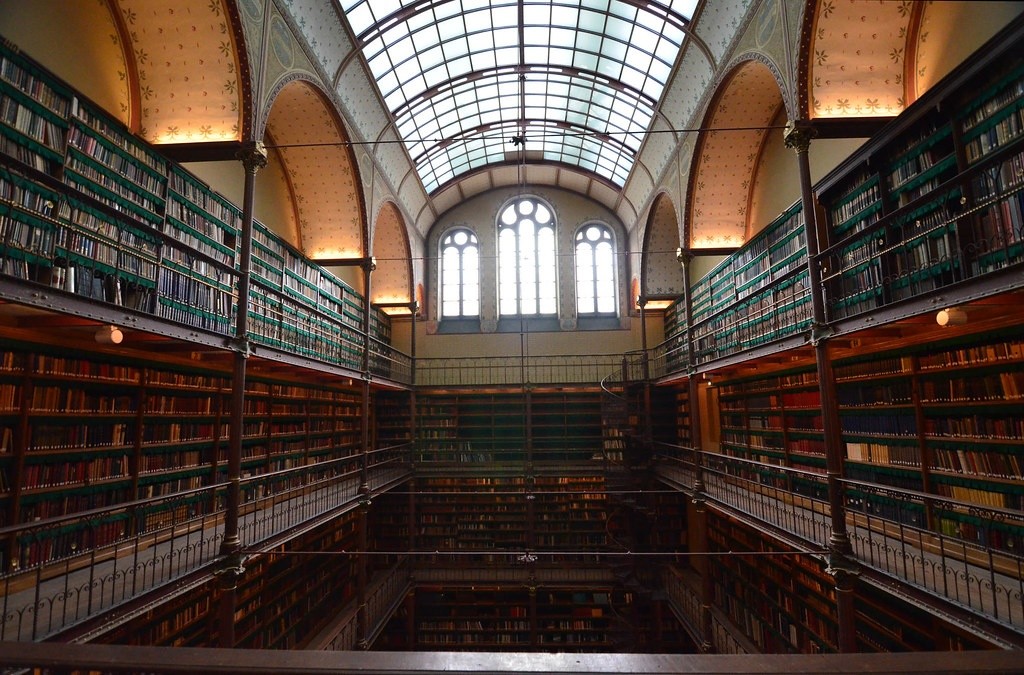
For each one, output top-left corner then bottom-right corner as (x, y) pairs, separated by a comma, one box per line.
(0, 57), (1024, 652)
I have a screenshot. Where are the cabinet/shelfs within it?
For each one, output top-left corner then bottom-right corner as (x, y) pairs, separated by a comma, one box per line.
(0, 35), (1024, 651)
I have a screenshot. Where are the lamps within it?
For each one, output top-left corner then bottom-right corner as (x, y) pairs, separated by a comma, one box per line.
(94, 324), (124, 344)
(935, 307), (968, 327)
